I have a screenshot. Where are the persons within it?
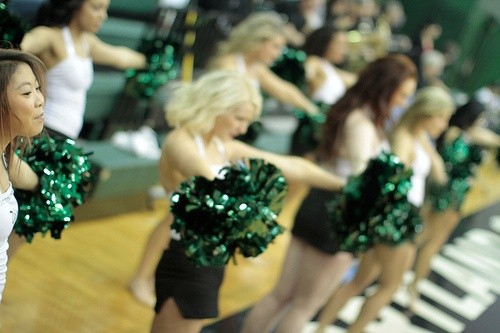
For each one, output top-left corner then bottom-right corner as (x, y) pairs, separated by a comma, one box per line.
(0, 48), (47, 302)
(416, 50), (448, 90)
(317, 86), (454, 333)
(128, 10), (324, 308)
(16, 0), (147, 146)
(240, 54), (418, 333)
(403, 101), (500, 298)
(283, 26), (360, 203)
(150, 69), (349, 333)
(278, 0), (500, 112)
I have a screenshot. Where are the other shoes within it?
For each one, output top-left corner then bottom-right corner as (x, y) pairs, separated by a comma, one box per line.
(128, 277), (154, 305)
